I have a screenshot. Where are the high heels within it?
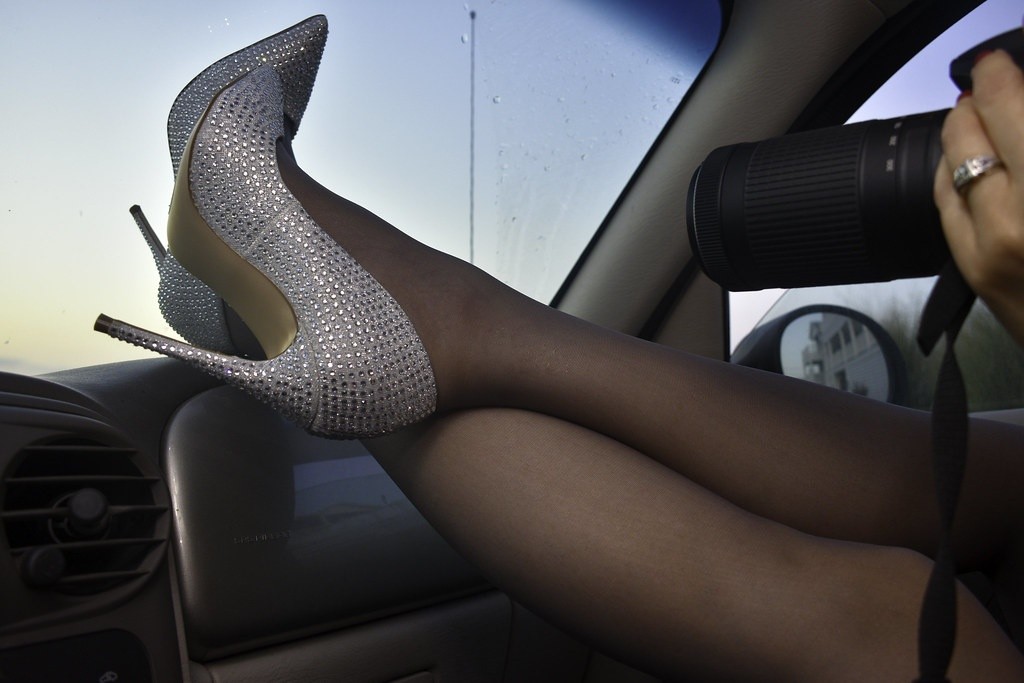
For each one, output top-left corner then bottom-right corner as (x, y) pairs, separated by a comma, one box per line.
(96, 65), (439, 439)
(126, 13), (330, 352)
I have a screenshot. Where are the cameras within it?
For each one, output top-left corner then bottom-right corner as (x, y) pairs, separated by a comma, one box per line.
(684, 23), (1024, 289)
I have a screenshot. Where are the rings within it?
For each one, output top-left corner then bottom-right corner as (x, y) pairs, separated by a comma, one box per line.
(953, 155), (1001, 189)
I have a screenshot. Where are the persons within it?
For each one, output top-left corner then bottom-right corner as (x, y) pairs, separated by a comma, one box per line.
(92, 15), (1024, 683)
